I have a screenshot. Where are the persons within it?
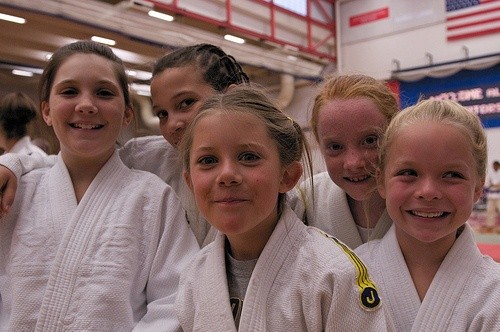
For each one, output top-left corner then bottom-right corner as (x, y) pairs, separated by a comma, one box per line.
(172, 81), (386, 332)
(0, 91), (47, 155)
(479, 160), (500, 228)
(353, 98), (500, 331)
(299, 74), (401, 250)
(0, 43), (305, 249)
(0, 40), (201, 332)
